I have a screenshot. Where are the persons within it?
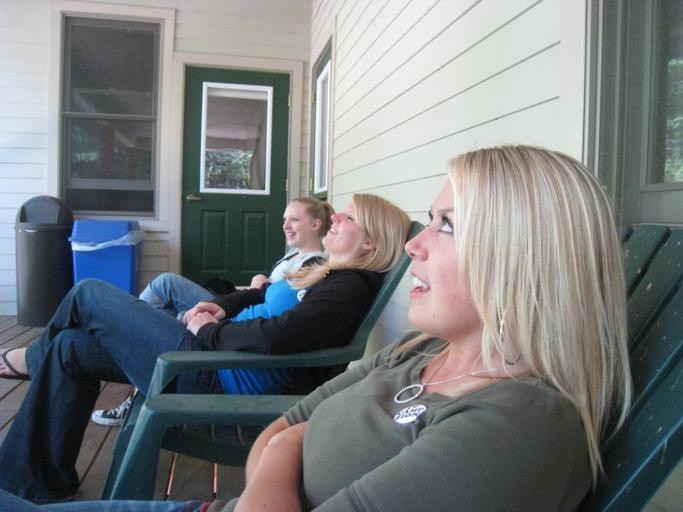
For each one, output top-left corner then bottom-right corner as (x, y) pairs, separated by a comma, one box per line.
(0, 192), (415, 506)
(91, 196), (336, 427)
(0, 144), (634, 512)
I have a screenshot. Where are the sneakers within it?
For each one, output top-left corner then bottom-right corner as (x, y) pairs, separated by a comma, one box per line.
(90, 393), (134, 426)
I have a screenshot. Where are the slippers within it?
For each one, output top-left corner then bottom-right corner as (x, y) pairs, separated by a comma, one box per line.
(0, 347), (33, 381)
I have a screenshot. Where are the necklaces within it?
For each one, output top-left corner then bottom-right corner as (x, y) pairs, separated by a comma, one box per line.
(394, 346), (523, 404)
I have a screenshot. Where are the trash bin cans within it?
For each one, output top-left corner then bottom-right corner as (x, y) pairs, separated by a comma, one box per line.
(68, 219), (142, 298)
(15, 195), (75, 327)
